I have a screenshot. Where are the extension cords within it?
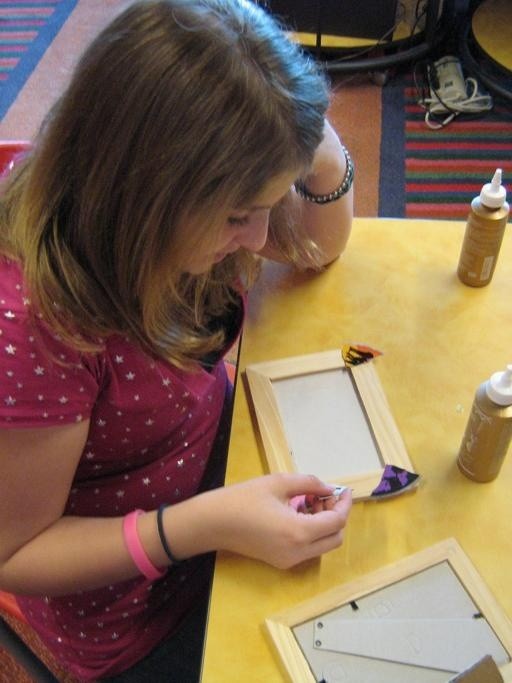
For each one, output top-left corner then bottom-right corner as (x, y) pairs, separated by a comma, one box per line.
(426, 55), (468, 115)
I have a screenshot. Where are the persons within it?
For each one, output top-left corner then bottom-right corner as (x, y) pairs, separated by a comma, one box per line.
(0, 0), (353, 683)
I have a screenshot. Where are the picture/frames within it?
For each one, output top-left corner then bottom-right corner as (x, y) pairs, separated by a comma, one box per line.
(242, 344), (419, 501)
(260, 535), (512, 681)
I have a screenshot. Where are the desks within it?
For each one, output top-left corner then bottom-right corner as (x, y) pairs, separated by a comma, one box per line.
(198, 211), (510, 682)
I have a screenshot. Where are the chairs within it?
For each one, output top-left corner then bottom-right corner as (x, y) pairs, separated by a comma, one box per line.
(0, 137), (50, 681)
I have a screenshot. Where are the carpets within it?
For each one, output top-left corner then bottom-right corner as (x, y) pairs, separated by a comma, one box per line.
(0, 1), (132, 145)
(379, 74), (512, 221)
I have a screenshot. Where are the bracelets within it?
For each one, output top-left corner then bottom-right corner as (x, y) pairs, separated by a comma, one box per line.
(294, 146), (354, 206)
(122, 508), (167, 581)
(155, 504), (179, 568)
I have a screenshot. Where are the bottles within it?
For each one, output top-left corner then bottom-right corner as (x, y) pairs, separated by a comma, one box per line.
(455, 362), (512, 483)
(455, 167), (511, 287)
